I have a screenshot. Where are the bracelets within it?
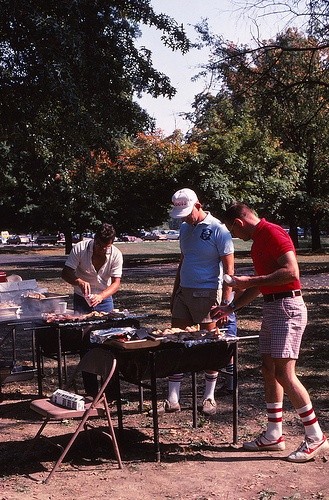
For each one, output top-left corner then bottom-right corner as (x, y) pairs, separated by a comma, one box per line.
(220, 299), (231, 305)
(229, 302), (239, 312)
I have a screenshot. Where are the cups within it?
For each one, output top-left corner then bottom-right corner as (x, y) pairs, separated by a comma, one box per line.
(58, 302), (66, 314)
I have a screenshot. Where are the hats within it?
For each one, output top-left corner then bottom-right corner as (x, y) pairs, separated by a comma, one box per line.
(169, 188), (198, 219)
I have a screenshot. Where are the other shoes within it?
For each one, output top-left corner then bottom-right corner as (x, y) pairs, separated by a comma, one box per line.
(217, 385), (235, 395)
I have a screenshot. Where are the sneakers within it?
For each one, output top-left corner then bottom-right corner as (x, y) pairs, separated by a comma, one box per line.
(148, 399), (181, 417)
(243, 430), (286, 451)
(288, 433), (329, 462)
(201, 397), (217, 416)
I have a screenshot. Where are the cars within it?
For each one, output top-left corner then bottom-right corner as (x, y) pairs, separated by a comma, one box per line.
(283, 227), (306, 236)
(6, 234), (30, 246)
(0, 228), (180, 245)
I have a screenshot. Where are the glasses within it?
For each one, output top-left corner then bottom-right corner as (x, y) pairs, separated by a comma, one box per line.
(229, 221), (235, 233)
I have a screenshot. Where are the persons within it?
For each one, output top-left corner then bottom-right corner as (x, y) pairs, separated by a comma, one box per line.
(61, 223), (129, 404)
(223, 203), (329, 463)
(148, 188), (234, 415)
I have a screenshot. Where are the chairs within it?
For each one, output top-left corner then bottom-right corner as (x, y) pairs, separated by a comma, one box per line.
(18, 348), (123, 484)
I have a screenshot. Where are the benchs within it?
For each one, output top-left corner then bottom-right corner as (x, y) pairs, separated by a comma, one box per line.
(35, 236), (58, 245)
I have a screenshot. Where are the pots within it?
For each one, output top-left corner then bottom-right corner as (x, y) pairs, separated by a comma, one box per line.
(20, 294), (69, 316)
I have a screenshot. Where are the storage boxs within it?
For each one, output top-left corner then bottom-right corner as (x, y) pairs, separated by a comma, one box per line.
(0, 271), (7, 283)
(51, 389), (85, 411)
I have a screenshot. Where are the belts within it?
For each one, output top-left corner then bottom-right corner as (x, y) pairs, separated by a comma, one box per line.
(263, 290), (301, 303)
